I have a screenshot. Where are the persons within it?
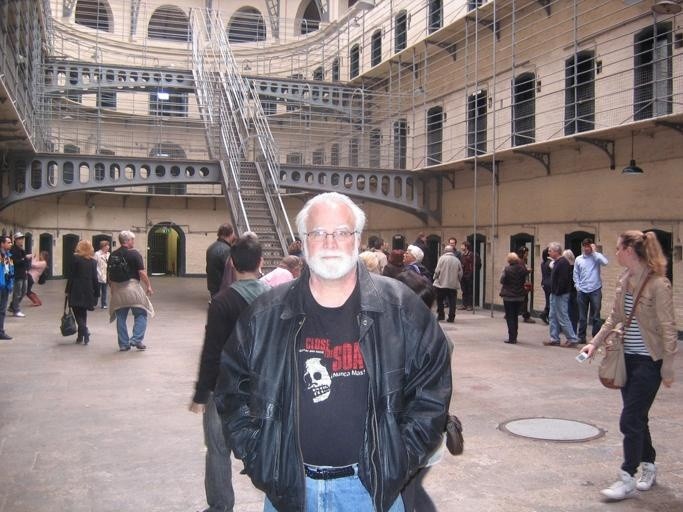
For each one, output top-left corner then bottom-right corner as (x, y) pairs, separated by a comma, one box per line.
(578, 231), (677, 499)
(106, 230), (154, 351)
(539, 242), (609, 349)
(220, 231), (257, 291)
(0, 235), (13, 339)
(189, 236), (271, 510)
(497, 246), (533, 346)
(9, 232), (31, 317)
(206, 223), (234, 295)
(360, 232), (434, 309)
(26, 250), (50, 306)
(258, 255), (303, 288)
(432, 238), (480, 321)
(215, 192), (453, 512)
(93, 240), (110, 309)
(64, 239), (100, 344)
(288, 241), (303, 255)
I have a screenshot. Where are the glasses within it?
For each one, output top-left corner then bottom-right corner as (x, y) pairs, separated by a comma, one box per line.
(304, 230), (357, 239)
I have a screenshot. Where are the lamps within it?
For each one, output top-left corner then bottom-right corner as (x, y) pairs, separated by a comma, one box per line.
(414, 52), (424, 93)
(620, 129), (644, 175)
(650, 0), (681, 14)
(87, 194), (97, 209)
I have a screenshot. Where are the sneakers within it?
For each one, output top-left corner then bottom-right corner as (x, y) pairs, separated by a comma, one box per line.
(120, 344), (130, 350)
(130, 337), (146, 349)
(561, 341), (577, 348)
(600, 470), (637, 499)
(14, 311), (25, 317)
(543, 339), (560, 346)
(637, 462), (657, 491)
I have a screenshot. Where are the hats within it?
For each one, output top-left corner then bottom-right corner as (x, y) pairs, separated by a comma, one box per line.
(14, 232), (25, 239)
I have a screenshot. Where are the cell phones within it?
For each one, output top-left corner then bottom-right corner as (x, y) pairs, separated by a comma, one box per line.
(575, 352), (588, 363)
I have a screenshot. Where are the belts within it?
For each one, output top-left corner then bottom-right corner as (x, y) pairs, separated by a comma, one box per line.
(304, 464), (355, 479)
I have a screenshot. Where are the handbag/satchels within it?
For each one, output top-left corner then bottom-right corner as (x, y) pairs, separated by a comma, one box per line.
(109, 255), (129, 281)
(598, 323), (628, 388)
(61, 314), (76, 335)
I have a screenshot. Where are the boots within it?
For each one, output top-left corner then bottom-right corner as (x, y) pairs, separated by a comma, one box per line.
(28, 293), (41, 306)
